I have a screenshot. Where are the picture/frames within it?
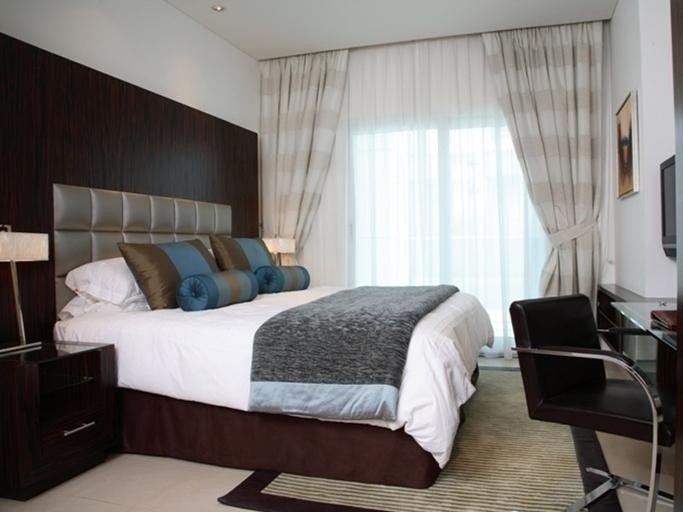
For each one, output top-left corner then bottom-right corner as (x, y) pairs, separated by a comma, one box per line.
(610, 83), (643, 203)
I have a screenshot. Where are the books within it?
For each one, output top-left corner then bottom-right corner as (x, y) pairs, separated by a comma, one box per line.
(649, 308), (677, 350)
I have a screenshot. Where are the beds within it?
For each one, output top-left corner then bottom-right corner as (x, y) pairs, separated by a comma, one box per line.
(42, 181), (497, 490)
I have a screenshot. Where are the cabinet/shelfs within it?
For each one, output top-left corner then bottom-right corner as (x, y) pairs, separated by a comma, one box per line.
(594, 280), (648, 356)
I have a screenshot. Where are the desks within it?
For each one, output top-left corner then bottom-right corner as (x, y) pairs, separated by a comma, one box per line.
(605, 295), (677, 363)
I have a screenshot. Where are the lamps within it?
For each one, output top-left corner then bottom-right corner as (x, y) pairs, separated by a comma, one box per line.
(260, 236), (298, 269)
(0, 229), (51, 355)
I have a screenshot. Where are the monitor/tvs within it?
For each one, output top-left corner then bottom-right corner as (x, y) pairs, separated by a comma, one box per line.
(660, 154), (677, 257)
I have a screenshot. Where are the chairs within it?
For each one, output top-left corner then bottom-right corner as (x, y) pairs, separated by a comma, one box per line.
(509, 291), (681, 510)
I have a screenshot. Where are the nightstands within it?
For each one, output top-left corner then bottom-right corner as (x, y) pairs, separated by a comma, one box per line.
(0, 333), (118, 503)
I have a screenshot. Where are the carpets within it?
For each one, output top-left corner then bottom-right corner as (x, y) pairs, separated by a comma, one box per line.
(215, 363), (628, 511)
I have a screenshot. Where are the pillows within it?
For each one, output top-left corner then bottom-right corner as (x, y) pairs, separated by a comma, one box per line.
(176, 264), (259, 314)
(253, 261), (313, 298)
(206, 232), (275, 279)
(63, 253), (156, 311)
(115, 236), (222, 317)
